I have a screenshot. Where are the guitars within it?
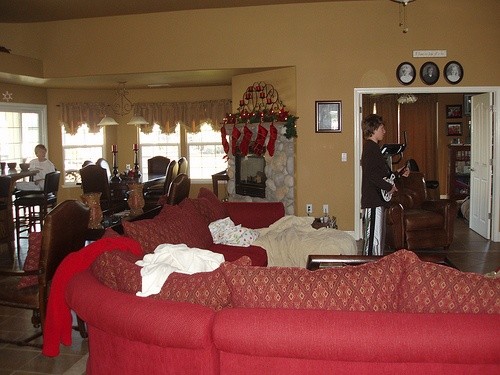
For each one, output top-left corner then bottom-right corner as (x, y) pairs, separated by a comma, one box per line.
(376, 162), (410, 203)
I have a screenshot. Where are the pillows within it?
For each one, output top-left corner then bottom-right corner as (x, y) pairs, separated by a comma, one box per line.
(91, 187), (500, 313)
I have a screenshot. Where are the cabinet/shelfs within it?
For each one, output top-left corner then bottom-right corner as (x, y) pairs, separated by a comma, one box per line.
(448, 144), (470, 196)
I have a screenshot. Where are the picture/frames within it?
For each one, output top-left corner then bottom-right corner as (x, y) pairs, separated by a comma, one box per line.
(463, 95), (472, 114)
(446, 122), (462, 136)
(395, 61), (464, 84)
(315, 100), (342, 133)
(445, 104), (463, 118)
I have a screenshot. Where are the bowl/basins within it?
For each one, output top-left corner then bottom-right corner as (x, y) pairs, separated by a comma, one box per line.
(19, 164), (30, 170)
(7, 163), (16, 169)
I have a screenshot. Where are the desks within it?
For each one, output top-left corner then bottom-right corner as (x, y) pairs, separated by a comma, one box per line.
(76, 173), (166, 210)
(0, 169), (39, 244)
(306, 254), (460, 274)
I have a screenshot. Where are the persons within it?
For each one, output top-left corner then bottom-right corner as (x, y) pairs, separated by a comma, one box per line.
(424, 66), (435, 82)
(401, 67), (413, 83)
(448, 65), (460, 82)
(450, 108), (460, 134)
(30, 145), (55, 185)
(361, 114), (410, 256)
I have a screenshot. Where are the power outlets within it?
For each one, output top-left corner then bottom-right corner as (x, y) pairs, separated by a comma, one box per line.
(306, 204), (313, 214)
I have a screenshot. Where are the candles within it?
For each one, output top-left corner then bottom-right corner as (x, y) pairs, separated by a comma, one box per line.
(111, 145), (117, 151)
(133, 143), (137, 149)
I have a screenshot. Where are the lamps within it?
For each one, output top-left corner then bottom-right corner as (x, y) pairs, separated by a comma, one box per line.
(396, 93), (418, 104)
(96, 81), (150, 126)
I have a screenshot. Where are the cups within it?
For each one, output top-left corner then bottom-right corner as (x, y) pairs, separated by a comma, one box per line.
(126, 164), (130, 170)
(1, 163), (5, 170)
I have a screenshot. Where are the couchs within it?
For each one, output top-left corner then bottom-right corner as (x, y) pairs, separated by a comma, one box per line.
(388, 180), (457, 252)
(62, 202), (500, 375)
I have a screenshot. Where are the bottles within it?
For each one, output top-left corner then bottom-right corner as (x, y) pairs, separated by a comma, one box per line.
(80, 192), (103, 229)
(128, 183), (145, 217)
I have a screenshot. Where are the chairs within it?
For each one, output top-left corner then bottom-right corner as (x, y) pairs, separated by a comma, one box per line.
(12, 156), (191, 335)
